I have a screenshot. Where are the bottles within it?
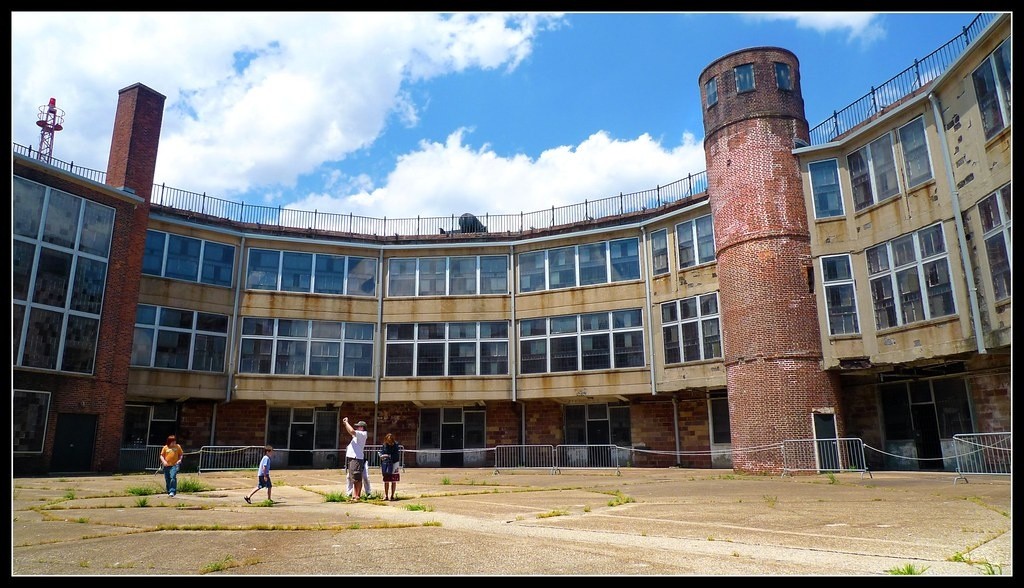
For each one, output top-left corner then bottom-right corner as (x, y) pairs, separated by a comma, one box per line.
(377, 451), (386, 461)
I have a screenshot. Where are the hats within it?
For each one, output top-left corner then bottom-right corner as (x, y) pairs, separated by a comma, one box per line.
(354, 421), (367, 427)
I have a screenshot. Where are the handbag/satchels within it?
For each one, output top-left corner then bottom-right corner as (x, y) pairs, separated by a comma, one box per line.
(391, 462), (400, 474)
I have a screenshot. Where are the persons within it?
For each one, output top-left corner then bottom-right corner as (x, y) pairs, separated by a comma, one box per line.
(244, 445), (277, 504)
(342, 417), (372, 504)
(377, 434), (400, 502)
(159, 435), (183, 498)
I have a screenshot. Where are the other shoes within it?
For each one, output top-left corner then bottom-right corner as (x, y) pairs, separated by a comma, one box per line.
(244, 496), (251, 504)
(380, 497), (388, 501)
(347, 497), (360, 503)
(270, 499), (277, 504)
(169, 491), (175, 497)
(392, 497), (394, 501)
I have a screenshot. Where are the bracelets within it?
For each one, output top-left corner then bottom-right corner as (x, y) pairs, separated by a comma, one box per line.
(178, 459), (181, 462)
